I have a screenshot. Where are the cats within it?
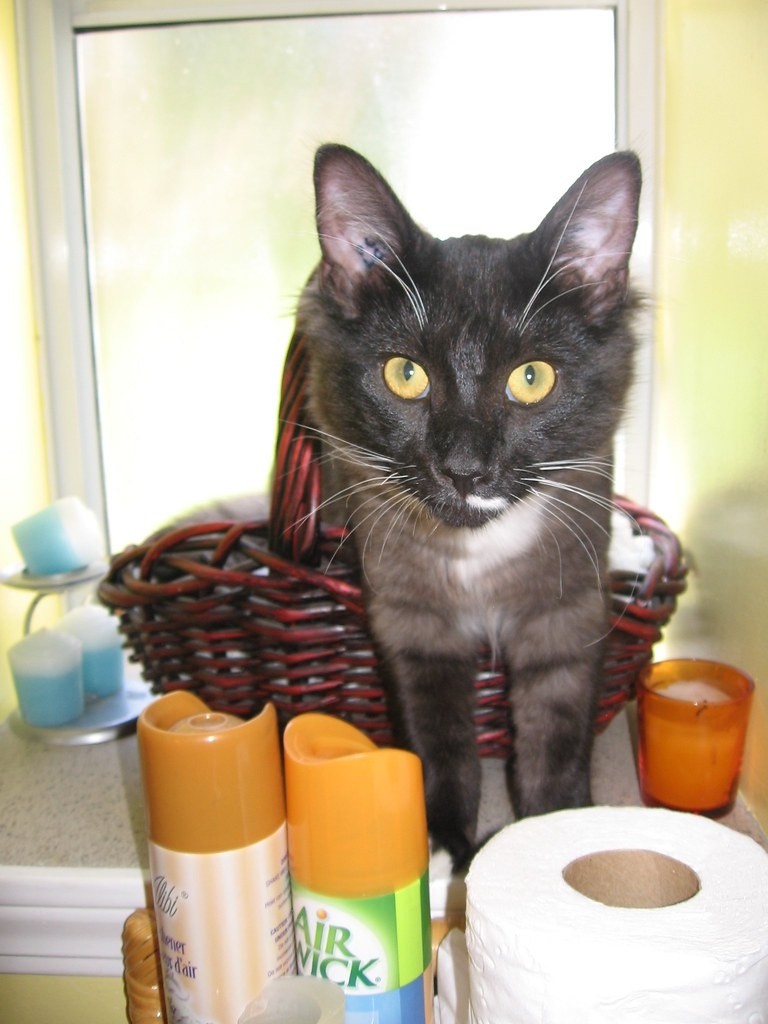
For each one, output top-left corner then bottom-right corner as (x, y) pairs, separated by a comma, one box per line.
(275, 143), (644, 874)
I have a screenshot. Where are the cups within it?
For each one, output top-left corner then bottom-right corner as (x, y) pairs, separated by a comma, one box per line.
(633, 657), (756, 819)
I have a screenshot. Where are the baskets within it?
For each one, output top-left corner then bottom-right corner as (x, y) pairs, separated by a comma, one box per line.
(95, 483), (694, 776)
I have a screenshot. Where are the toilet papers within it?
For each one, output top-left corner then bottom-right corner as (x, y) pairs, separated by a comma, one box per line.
(464, 804), (767, 1024)
